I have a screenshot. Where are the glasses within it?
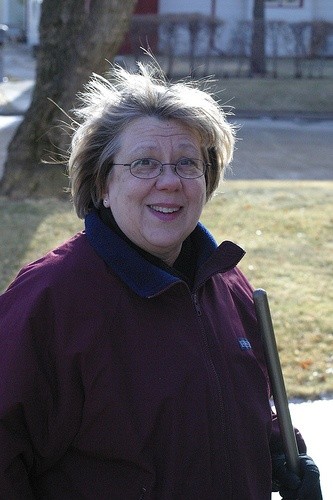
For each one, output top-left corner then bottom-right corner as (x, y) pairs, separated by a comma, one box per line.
(109, 157), (212, 180)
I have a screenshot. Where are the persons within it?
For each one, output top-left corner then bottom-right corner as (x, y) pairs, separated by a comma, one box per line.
(1, 45), (323, 500)
